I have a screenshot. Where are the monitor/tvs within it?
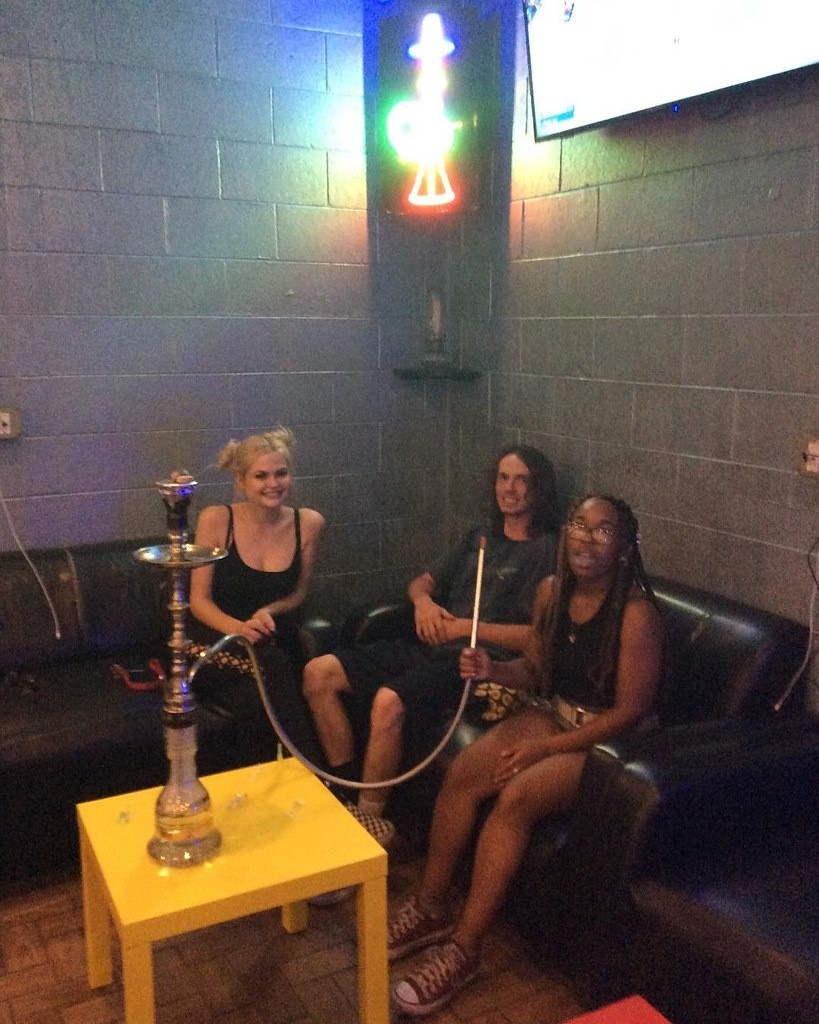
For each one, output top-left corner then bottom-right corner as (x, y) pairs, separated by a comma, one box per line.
(523, 0), (819, 143)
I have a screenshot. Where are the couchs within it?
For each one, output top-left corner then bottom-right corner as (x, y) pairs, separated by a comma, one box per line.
(355, 572), (819, 1024)
(0, 514), (339, 875)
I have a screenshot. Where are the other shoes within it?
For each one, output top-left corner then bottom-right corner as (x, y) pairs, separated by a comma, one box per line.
(311, 887), (353, 904)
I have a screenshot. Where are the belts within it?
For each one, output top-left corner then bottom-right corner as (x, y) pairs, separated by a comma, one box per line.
(554, 695), (601, 728)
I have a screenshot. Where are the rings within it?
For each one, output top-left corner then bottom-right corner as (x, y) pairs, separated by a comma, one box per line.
(513, 765), (522, 775)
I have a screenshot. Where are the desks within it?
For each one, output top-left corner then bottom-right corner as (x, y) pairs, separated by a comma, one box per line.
(76, 756), (390, 1024)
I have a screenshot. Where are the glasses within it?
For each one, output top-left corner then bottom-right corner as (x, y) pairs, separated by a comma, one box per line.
(567, 521), (618, 544)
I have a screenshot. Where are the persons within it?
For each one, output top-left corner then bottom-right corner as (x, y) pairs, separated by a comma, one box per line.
(188, 426), (396, 846)
(386, 494), (666, 1015)
(303, 447), (554, 907)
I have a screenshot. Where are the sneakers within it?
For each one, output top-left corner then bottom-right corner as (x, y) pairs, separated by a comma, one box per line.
(392, 933), (483, 1016)
(386, 895), (457, 958)
